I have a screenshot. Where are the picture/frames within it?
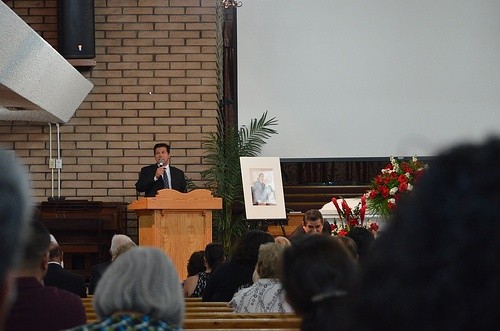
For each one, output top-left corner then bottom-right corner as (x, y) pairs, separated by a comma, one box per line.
(239, 155), (287, 220)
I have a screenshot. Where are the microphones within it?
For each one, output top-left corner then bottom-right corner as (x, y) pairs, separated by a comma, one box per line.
(157, 159), (164, 181)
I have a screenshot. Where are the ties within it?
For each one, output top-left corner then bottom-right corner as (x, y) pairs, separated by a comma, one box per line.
(163, 168), (169, 189)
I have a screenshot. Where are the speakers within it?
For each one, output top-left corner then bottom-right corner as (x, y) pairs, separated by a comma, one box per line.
(57, 0), (96, 59)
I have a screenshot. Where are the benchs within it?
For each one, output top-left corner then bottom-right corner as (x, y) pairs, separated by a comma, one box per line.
(81, 294), (303, 331)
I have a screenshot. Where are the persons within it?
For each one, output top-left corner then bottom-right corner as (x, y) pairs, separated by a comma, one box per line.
(1, 220), (87, 331)
(202, 229), (274, 301)
(58, 246), (185, 330)
(42, 242), (86, 299)
(180, 250), (206, 288)
(1, 148), (34, 329)
(134, 143), (187, 197)
(253, 172), (268, 202)
(288, 210), (332, 246)
(280, 232), (363, 331)
(88, 234), (137, 295)
(336, 236), (359, 263)
(345, 226), (375, 254)
(182, 243), (227, 298)
(228, 242), (296, 314)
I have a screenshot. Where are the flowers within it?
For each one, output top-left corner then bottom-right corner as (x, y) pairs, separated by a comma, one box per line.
(327, 195), (380, 239)
(364, 154), (428, 222)
